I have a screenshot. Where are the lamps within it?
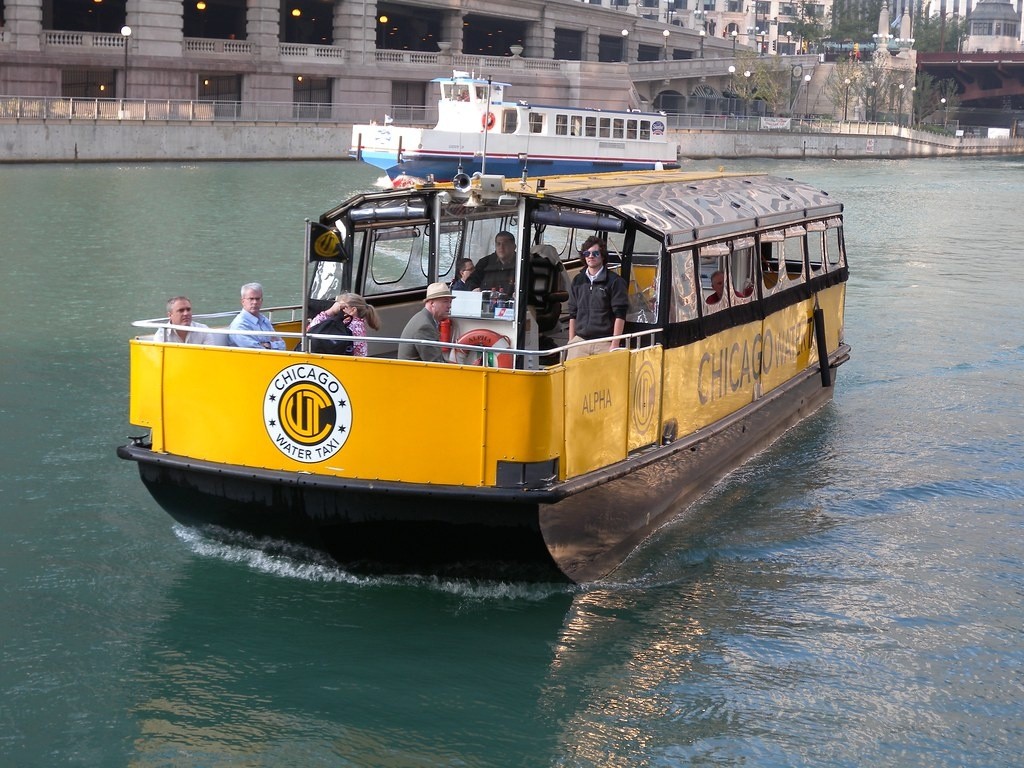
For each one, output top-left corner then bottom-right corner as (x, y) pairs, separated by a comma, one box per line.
(437, 190), (451, 204)
(496, 192), (518, 206)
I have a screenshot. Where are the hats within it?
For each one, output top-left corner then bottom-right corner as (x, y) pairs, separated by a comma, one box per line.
(423, 282), (456, 303)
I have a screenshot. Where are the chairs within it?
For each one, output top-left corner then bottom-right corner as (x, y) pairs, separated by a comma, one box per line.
(527, 245), (568, 366)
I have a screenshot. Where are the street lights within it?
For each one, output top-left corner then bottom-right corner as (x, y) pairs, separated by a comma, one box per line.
(664, 0), (674, 23)
(744, 71), (751, 118)
(787, 30), (792, 55)
(121, 26), (132, 98)
(700, 30), (705, 58)
(728, 66), (735, 115)
(845, 79), (851, 120)
(805, 75), (811, 118)
(941, 98), (946, 110)
(899, 84), (904, 122)
(760, 31), (765, 56)
(621, 29), (628, 61)
(732, 31), (737, 57)
(663, 30), (670, 60)
(871, 81), (877, 121)
(911, 86), (916, 125)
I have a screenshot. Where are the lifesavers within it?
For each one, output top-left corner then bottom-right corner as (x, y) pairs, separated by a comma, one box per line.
(482, 112), (496, 130)
(447, 328), (514, 370)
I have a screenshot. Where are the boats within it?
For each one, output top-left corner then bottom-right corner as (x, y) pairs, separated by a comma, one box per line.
(116, 166), (851, 582)
(349, 69), (682, 189)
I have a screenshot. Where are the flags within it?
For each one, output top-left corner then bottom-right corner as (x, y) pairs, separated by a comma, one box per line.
(307, 221), (350, 263)
(385, 116), (393, 123)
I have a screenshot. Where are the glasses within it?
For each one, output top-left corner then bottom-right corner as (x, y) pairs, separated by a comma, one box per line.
(583, 251), (599, 257)
(464, 268), (473, 272)
(244, 297), (263, 303)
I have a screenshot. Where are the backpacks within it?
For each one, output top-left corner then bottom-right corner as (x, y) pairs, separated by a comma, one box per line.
(293, 308), (354, 356)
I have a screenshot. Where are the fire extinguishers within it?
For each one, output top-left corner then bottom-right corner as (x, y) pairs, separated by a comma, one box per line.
(439, 319), (451, 352)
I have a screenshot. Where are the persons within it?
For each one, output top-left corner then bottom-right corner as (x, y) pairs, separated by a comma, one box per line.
(456, 90), (484, 102)
(705, 270), (744, 305)
(465, 231), (537, 329)
(565, 236), (629, 361)
(226, 283), (286, 351)
(307, 293), (379, 357)
(397, 283), (458, 364)
(453, 258), (475, 290)
(797, 50), (861, 62)
(155, 296), (215, 344)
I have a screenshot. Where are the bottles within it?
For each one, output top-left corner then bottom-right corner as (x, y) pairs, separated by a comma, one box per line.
(489, 288), (506, 312)
